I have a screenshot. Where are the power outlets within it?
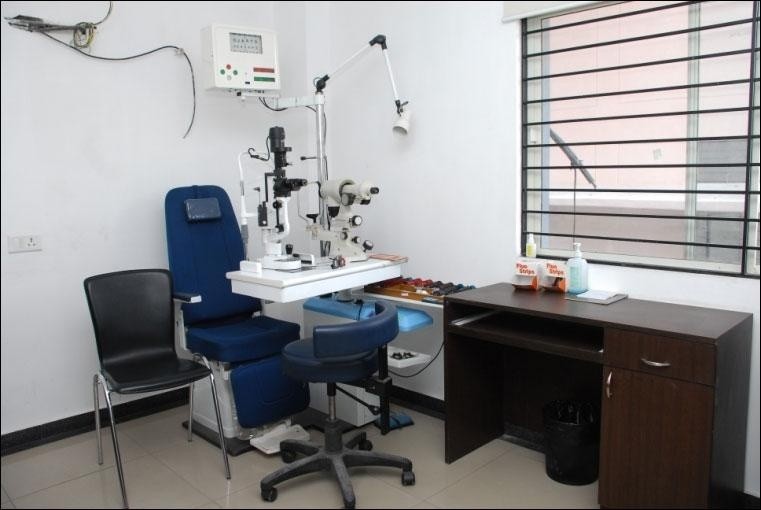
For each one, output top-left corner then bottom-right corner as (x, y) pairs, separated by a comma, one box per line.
(23, 234), (41, 252)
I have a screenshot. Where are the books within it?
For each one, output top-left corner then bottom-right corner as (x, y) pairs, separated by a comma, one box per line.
(576, 287), (628, 305)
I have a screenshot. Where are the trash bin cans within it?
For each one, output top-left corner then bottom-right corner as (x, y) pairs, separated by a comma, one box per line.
(545, 400), (600, 485)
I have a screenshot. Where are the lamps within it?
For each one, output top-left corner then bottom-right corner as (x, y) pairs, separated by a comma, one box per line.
(314, 31), (411, 137)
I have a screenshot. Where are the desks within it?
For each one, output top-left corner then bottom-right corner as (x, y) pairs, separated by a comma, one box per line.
(224, 252), (407, 305)
(441, 284), (754, 509)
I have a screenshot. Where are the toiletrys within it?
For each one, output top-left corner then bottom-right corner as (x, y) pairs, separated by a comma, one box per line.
(566, 242), (589, 293)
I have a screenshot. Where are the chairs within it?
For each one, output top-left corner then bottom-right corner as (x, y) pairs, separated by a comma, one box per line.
(257, 300), (414, 508)
(163, 184), (312, 461)
(84, 268), (231, 508)
